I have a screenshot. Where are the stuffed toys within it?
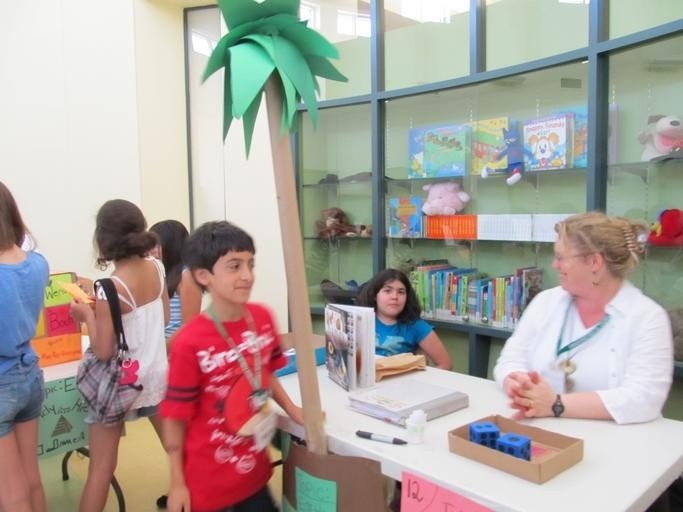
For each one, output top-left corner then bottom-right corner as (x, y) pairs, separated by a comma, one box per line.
(638, 115), (683, 162)
(646, 208), (683, 247)
(420, 181), (470, 218)
(481, 120), (540, 185)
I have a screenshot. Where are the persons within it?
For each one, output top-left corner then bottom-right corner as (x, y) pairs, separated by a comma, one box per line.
(68, 197), (171, 512)
(147, 219), (202, 363)
(0, 181), (50, 512)
(355, 267), (452, 373)
(159, 219), (326, 512)
(492, 209), (675, 426)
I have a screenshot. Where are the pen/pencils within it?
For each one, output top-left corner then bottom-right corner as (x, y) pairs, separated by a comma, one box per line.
(356, 430), (408, 445)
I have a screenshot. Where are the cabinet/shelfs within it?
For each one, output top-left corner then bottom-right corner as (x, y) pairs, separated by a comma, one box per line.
(294, 18), (683, 378)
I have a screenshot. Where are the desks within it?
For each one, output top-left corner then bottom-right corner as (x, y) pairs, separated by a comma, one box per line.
(37, 331), (129, 512)
(269, 351), (683, 511)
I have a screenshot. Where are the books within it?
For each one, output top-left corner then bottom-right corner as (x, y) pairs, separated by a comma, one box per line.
(413, 248), (543, 331)
(347, 378), (469, 429)
(324, 303), (376, 394)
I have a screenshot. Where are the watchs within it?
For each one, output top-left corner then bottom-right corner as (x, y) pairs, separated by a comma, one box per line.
(552, 394), (564, 418)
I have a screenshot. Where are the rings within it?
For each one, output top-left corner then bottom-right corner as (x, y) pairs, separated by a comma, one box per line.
(529, 398), (535, 408)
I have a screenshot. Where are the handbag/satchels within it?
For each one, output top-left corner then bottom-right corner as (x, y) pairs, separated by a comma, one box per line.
(75, 278), (143, 424)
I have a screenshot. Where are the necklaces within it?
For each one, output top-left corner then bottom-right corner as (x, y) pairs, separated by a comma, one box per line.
(559, 300), (615, 391)
